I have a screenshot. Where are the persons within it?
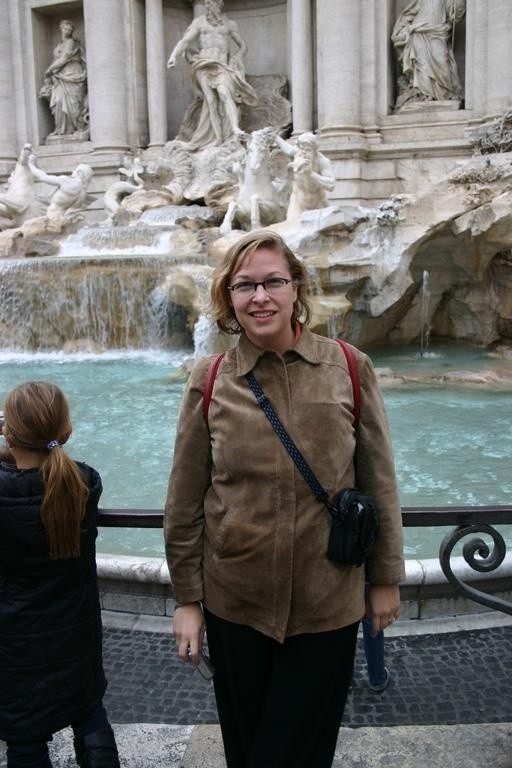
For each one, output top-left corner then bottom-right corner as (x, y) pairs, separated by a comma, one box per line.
(39, 21), (89, 134)
(0, 381), (120, 767)
(273, 133), (335, 220)
(167, 0), (247, 150)
(28, 155), (93, 223)
(391, 0), (465, 101)
(164, 230), (405, 767)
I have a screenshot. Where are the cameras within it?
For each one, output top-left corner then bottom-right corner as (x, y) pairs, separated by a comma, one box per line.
(188, 650), (217, 680)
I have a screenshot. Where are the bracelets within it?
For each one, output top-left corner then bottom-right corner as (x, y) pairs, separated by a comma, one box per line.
(174, 602), (194, 611)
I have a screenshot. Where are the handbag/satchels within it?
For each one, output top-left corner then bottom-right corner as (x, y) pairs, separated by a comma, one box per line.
(325, 485), (380, 572)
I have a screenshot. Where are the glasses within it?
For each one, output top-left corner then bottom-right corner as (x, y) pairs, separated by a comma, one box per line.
(225, 277), (294, 298)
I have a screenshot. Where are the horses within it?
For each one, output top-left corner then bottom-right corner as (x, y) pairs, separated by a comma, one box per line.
(219, 127), (294, 236)
(1, 143), (99, 227)
